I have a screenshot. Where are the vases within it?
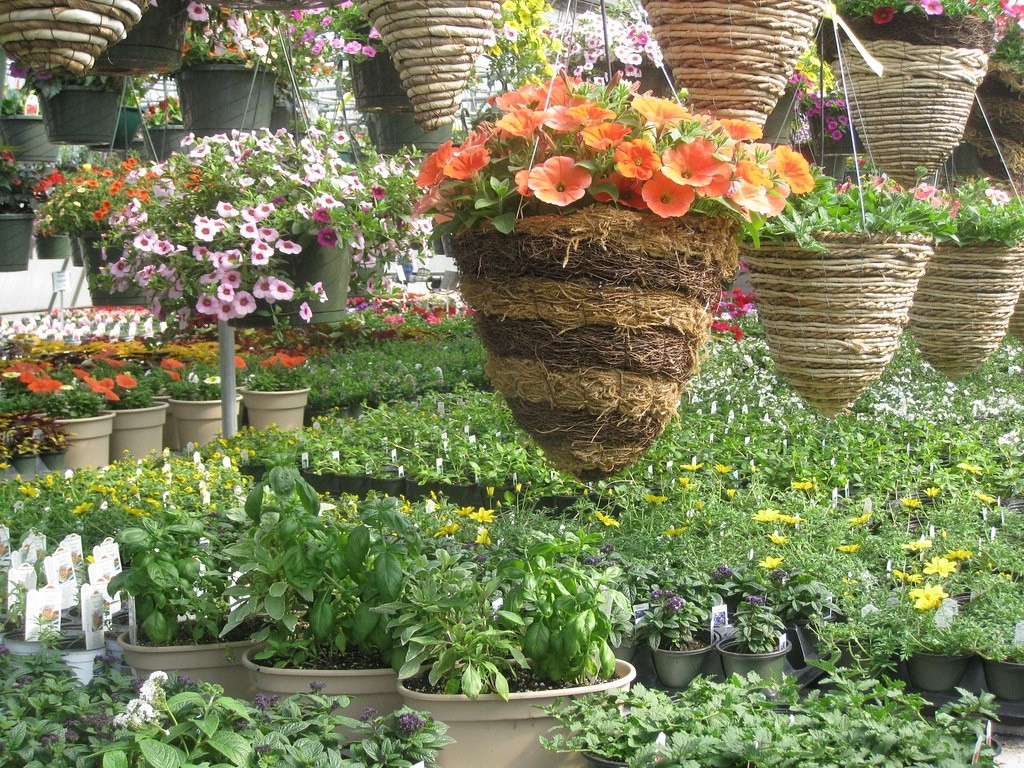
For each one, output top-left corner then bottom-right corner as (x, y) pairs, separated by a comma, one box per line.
(0, 213), (36, 272)
(81, 231), (158, 304)
(117, 630), (256, 702)
(149, 126), (185, 163)
(173, 62), (279, 136)
(351, 45), (411, 109)
(365, 110), (453, 154)
(41, 413), (113, 472)
(1, 114), (62, 162)
(166, 396), (244, 447)
(975, 651), (1024, 701)
(51, 640), (106, 688)
(395, 658), (637, 768)
(230, 231), (353, 328)
(906, 647), (975, 692)
(36, 84), (119, 145)
(89, 1), (187, 74)
(650, 628), (719, 687)
(104, 403), (169, 464)
(716, 635), (792, 684)
(240, 387), (309, 430)
(35, 232), (68, 258)
(240, 643), (402, 744)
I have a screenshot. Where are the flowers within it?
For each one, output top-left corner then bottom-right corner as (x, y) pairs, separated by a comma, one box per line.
(0, 1), (1023, 768)
